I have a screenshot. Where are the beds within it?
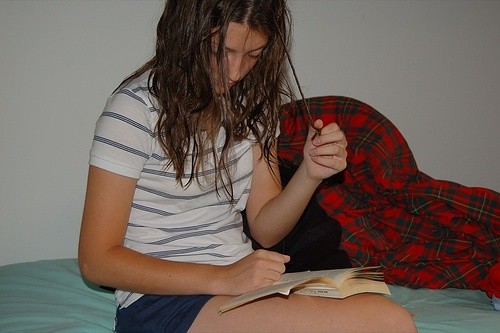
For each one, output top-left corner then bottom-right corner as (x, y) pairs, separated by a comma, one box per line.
(0, 95), (500, 333)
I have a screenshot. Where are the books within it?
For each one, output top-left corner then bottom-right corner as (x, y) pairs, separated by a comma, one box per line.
(217, 261), (391, 314)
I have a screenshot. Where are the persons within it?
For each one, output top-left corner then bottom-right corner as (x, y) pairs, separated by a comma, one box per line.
(79, 1), (418, 333)
